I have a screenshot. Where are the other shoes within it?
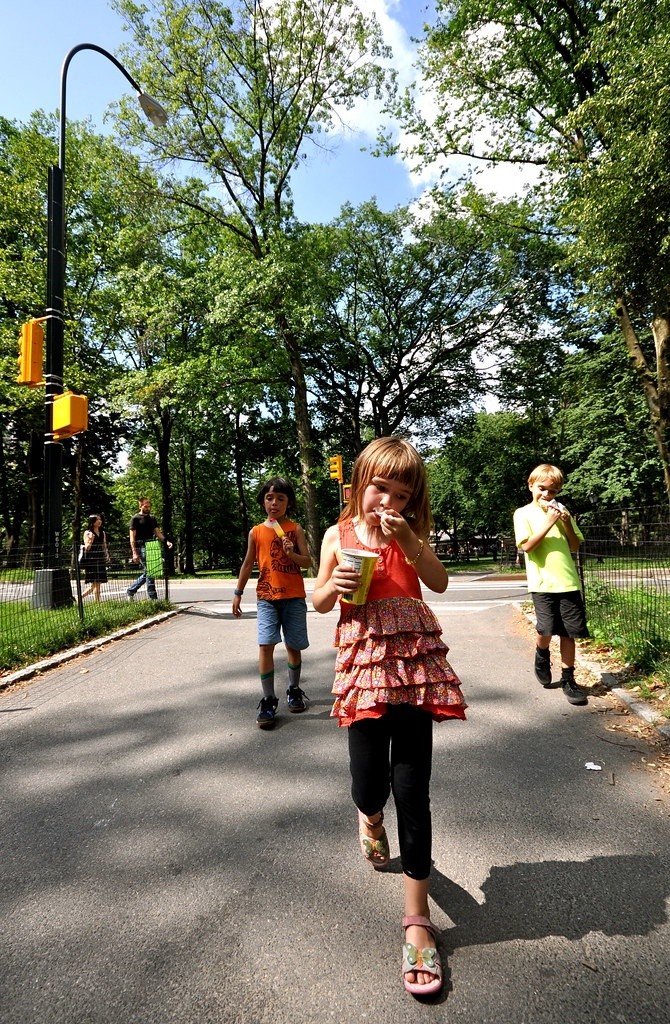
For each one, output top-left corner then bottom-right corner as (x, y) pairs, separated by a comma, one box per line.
(126, 590), (134, 603)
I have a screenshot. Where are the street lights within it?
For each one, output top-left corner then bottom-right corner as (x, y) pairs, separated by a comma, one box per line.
(30, 43), (169, 610)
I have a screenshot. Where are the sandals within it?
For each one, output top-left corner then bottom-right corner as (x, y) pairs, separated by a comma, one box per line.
(357, 808), (390, 867)
(401, 915), (443, 995)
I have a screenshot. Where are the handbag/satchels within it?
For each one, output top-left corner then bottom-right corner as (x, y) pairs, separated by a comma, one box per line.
(78, 544), (86, 569)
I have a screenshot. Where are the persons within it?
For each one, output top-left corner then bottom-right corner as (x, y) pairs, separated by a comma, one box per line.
(77, 514), (110, 601)
(514, 464), (590, 704)
(127, 497), (172, 602)
(311, 437), (468, 993)
(233, 478), (312, 726)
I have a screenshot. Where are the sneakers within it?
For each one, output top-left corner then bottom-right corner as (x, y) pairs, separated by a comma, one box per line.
(560, 675), (587, 703)
(534, 650), (552, 685)
(286, 682), (309, 712)
(256, 695), (279, 725)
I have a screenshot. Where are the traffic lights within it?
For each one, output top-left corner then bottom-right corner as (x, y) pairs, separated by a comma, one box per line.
(16, 324), (42, 385)
(341, 484), (353, 505)
(330, 455), (343, 482)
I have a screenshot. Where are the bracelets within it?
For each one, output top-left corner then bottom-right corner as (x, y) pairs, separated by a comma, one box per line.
(234, 590), (243, 595)
(405, 539), (424, 564)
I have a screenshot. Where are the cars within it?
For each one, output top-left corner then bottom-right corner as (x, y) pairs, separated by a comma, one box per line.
(435, 528), (503, 555)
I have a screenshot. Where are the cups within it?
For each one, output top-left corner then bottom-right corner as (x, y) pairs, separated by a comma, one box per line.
(339, 549), (380, 605)
